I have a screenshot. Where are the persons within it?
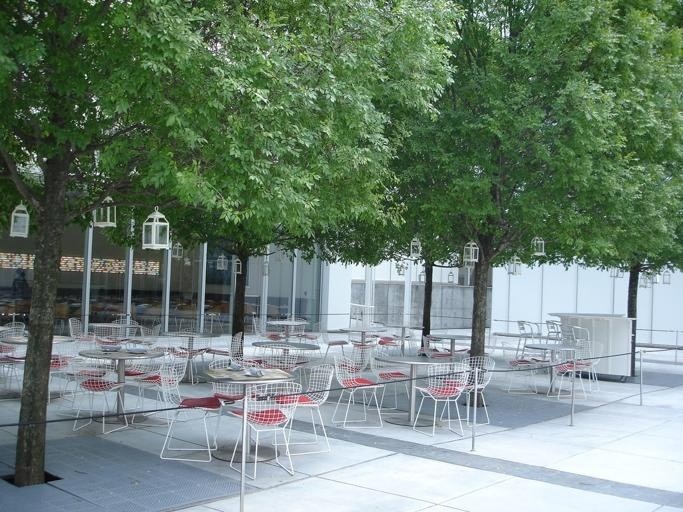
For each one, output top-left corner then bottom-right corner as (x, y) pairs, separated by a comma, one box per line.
(11, 267), (30, 299)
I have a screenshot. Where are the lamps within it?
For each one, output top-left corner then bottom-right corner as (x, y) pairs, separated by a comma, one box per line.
(173, 241), (184, 260)
(9, 200), (30, 237)
(233, 257), (242, 274)
(217, 254), (228, 270)
(93, 195), (116, 228)
(142, 205), (169, 251)
(410, 236), (671, 288)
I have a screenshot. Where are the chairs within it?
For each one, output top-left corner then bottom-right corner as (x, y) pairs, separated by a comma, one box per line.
(2, 299), (600, 437)
(272, 364), (334, 457)
(230, 382), (297, 479)
(156, 366), (218, 463)
